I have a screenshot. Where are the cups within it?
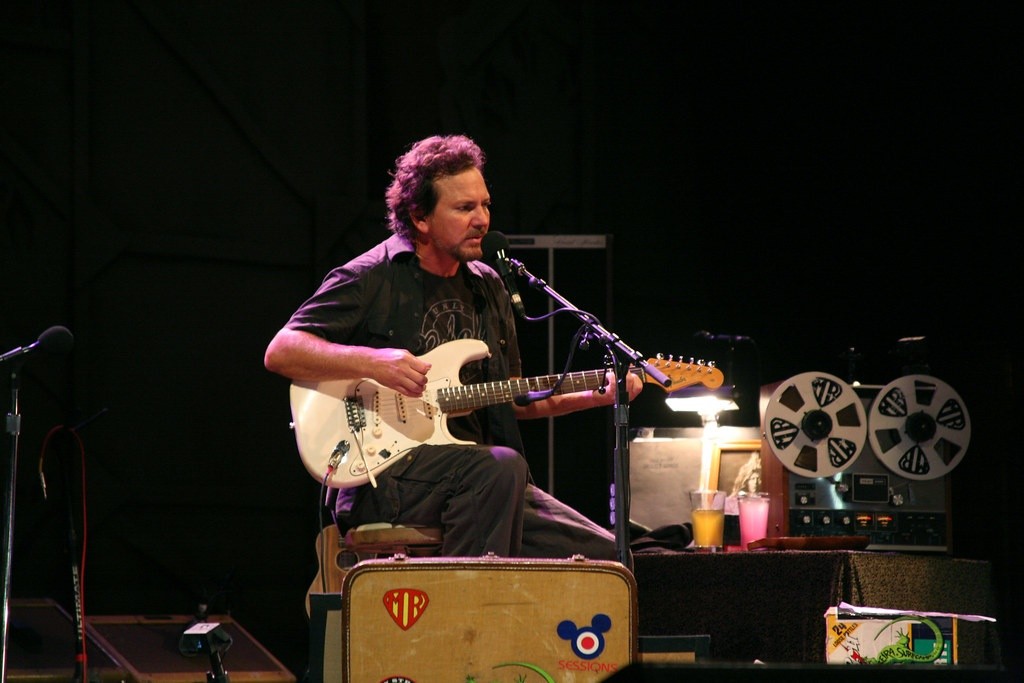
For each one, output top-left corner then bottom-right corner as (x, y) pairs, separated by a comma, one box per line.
(736, 493), (770, 551)
(689, 490), (727, 553)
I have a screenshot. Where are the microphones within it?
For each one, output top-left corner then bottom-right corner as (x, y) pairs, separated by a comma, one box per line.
(481, 231), (525, 317)
(514, 390), (555, 407)
(179, 621), (230, 657)
(0, 326), (74, 373)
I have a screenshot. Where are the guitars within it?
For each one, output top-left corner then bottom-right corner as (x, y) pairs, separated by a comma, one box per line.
(286, 336), (726, 492)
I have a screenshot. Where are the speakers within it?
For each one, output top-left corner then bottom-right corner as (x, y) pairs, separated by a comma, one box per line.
(3, 595), (297, 683)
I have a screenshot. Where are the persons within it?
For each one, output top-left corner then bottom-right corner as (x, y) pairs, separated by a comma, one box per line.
(264, 135), (644, 574)
